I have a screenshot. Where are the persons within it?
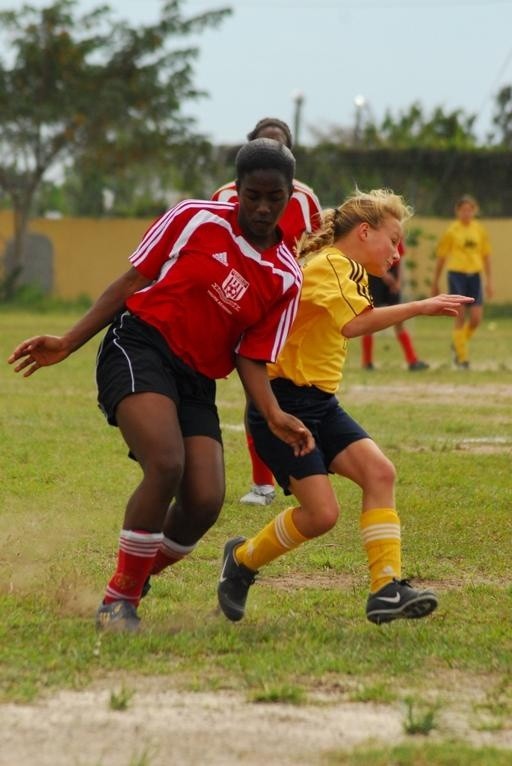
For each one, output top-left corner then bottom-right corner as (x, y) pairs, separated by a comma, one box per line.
(431, 197), (493, 370)
(210, 118), (323, 506)
(219, 188), (476, 623)
(9, 137), (316, 632)
(362, 236), (429, 372)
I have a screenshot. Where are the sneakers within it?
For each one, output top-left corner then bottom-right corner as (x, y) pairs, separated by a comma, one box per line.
(409, 361), (429, 371)
(240, 484), (277, 507)
(365, 577), (439, 624)
(95, 599), (141, 635)
(216, 534), (260, 622)
